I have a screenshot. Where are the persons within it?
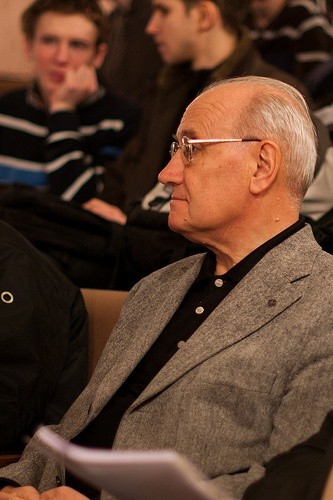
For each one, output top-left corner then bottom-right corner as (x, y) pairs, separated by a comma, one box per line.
(0, 220), (90, 473)
(0, 0), (328, 292)
(235, 0), (333, 83)
(95, 0), (332, 255)
(0, 76), (333, 500)
(0, 0), (137, 208)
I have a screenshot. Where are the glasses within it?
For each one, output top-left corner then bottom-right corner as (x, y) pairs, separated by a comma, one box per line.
(169, 136), (262, 167)
